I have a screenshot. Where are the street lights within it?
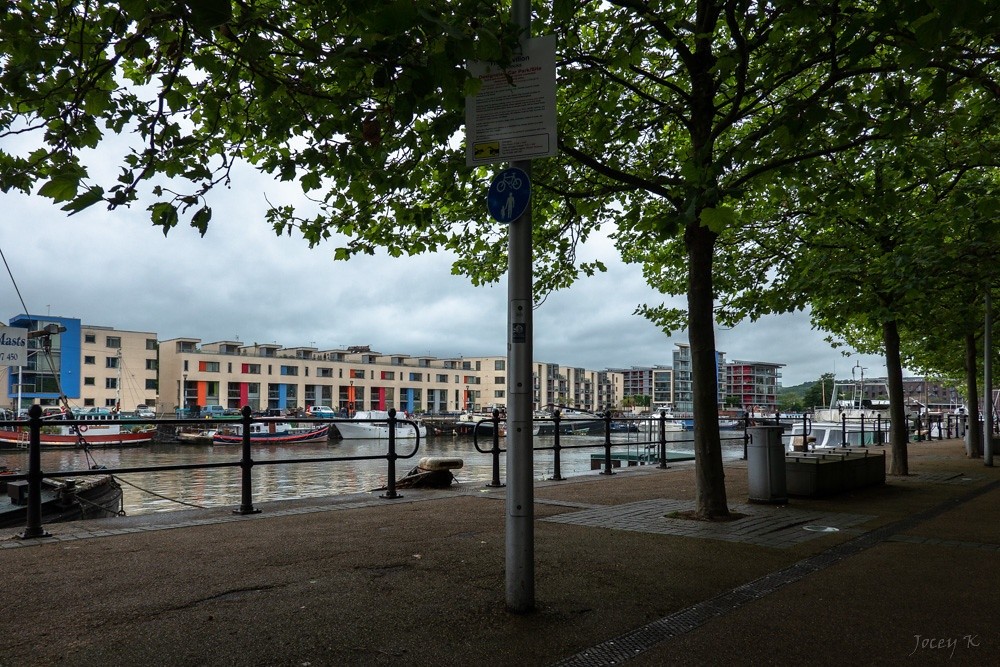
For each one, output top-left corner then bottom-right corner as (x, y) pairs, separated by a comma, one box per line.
(183, 374), (188, 409)
(466, 386), (469, 413)
(350, 381), (353, 418)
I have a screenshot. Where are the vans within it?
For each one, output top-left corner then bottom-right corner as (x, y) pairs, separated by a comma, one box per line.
(309, 406), (334, 417)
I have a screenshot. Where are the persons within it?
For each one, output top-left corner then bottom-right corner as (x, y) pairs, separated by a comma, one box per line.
(341, 406), (347, 418)
(5, 407), (12, 420)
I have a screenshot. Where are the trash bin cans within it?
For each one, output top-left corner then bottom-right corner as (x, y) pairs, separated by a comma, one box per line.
(746, 426), (787, 502)
(964, 421), (984, 454)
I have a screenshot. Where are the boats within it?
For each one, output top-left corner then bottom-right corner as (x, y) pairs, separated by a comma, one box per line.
(177, 416), (329, 445)
(0, 249), (123, 526)
(0, 413), (153, 448)
(454, 406), (638, 439)
(631, 406), (682, 432)
(682, 415), (740, 430)
(788, 360), (984, 451)
(332, 409), (427, 439)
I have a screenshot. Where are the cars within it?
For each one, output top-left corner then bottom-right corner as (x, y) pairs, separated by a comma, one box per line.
(133, 409), (156, 418)
(225, 408), (241, 415)
(88, 407), (111, 412)
(200, 405), (225, 416)
(43, 408), (62, 420)
